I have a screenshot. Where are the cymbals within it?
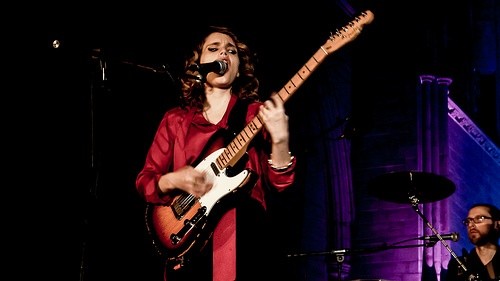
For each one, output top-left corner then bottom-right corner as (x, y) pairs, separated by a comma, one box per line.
(369, 167), (456, 205)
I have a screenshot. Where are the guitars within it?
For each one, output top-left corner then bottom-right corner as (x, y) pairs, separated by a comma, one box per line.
(143, 10), (375, 268)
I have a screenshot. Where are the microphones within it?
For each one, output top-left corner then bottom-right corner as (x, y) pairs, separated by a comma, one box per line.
(420, 232), (460, 242)
(189, 59), (227, 76)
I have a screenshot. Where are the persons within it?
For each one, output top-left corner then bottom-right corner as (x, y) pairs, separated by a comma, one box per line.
(134, 27), (295, 280)
(446, 204), (500, 280)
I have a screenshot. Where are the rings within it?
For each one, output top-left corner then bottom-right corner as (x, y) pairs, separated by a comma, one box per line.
(193, 178), (197, 186)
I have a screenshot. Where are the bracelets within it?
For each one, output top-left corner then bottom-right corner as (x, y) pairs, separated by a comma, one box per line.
(268, 152), (295, 171)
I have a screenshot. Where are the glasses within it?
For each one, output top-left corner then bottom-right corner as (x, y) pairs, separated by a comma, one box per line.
(463, 216), (492, 226)
